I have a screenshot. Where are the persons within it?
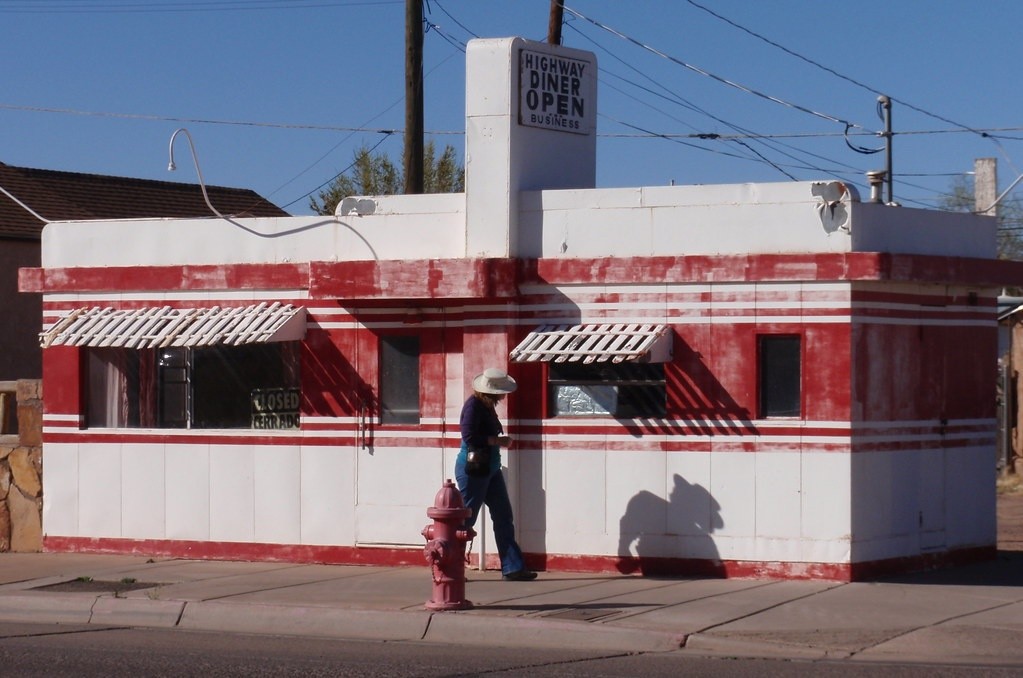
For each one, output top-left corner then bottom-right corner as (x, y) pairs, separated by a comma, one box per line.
(454, 368), (538, 581)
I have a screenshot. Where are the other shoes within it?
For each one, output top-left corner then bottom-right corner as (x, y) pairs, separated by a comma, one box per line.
(502, 567), (537, 580)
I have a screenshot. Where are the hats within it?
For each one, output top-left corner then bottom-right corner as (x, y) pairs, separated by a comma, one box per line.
(471, 368), (517, 394)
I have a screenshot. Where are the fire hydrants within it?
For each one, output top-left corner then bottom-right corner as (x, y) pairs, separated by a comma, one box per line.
(421, 478), (478, 610)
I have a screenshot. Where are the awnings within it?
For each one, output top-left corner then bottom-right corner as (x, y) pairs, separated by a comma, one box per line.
(507, 324), (673, 363)
(37, 303), (310, 344)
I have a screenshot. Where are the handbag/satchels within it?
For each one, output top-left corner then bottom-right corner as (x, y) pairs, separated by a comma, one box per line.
(464, 444), (491, 478)
